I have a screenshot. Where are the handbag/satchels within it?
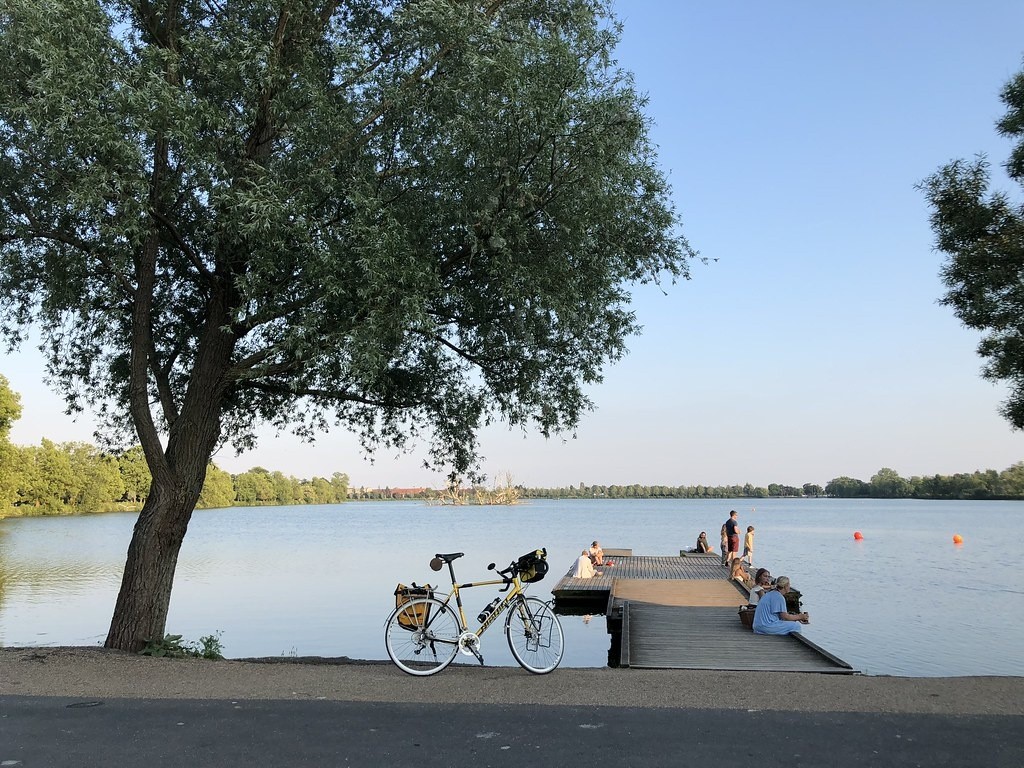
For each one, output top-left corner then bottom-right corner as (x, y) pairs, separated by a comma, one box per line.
(686, 546), (691, 553)
(738, 603), (758, 629)
(518, 550), (547, 583)
(393, 582), (434, 631)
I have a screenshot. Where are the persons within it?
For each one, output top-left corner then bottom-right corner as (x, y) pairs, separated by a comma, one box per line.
(752, 576), (810, 635)
(589, 541), (604, 566)
(730, 557), (750, 582)
(725, 510), (742, 566)
(748, 568), (770, 605)
(743, 573), (776, 589)
(739, 526), (755, 568)
(720, 524), (728, 566)
(697, 532), (713, 554)
(573, 551), (603, 579)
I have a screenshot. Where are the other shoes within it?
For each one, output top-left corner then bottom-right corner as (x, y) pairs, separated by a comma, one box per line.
(749, 566), (754, 568)
(597, 564), (603, 566)
(725, 565), (730, 568)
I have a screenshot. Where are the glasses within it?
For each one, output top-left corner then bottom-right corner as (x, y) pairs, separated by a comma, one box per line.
(762, 573), (771, 578)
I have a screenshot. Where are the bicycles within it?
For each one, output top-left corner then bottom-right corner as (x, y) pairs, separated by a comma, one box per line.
(383, 547), (565, 677)
